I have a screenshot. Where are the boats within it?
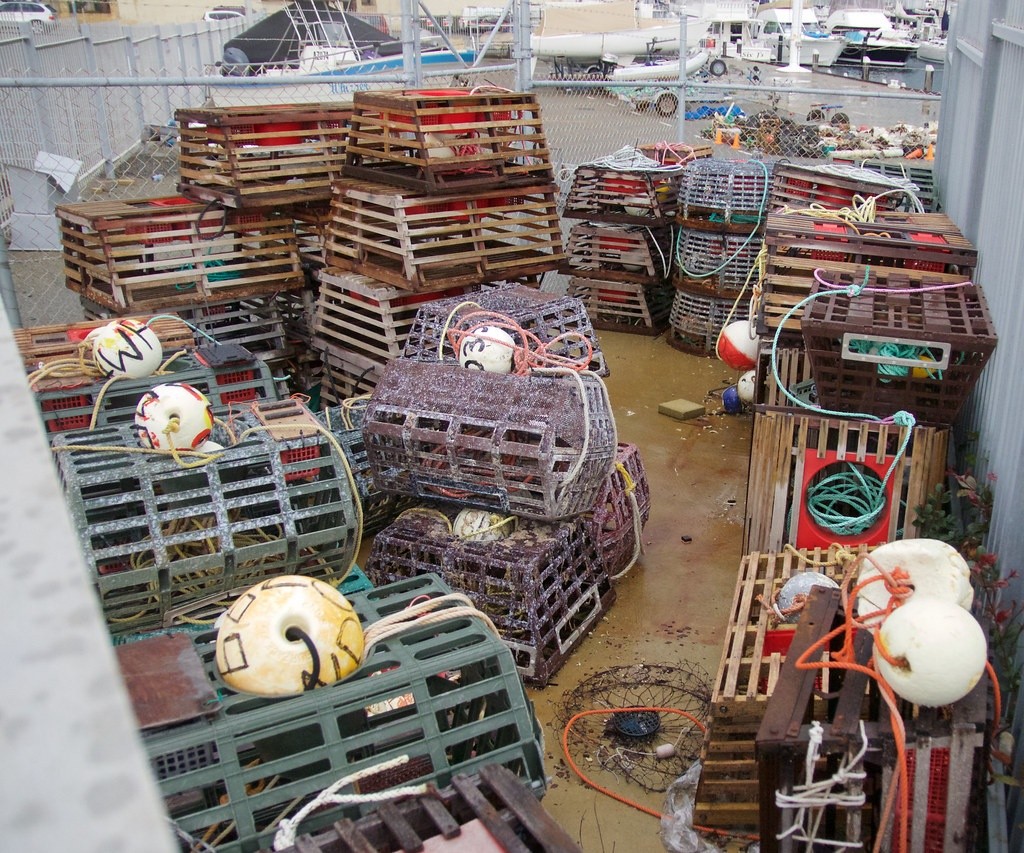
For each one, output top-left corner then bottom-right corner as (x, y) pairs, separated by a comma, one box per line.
(675, 0), (947, 83)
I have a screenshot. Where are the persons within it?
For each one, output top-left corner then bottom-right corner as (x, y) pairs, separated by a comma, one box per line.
(449, 75), (461, 87)
(752, 66), (761, 83)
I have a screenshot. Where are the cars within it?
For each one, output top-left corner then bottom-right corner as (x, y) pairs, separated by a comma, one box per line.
(0, 1), (56, 34)
(203, 10), (244, 22)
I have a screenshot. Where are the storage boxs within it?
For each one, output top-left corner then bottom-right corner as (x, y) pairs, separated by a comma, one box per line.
(15, 81), (1024, 851)
